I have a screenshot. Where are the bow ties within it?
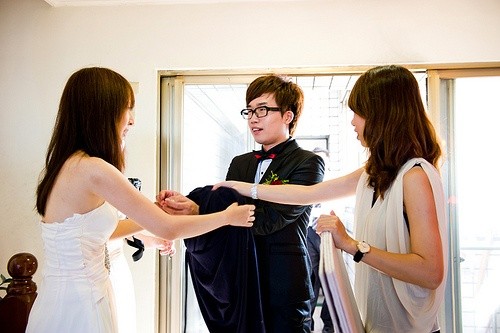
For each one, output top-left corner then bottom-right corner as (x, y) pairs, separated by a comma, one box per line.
(253, 150), (276, 162)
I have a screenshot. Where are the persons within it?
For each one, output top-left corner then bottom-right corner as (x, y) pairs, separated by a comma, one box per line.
(156, 74), (325, 333)
(211, 65), (448, 333)
(24, 66), (256, 333)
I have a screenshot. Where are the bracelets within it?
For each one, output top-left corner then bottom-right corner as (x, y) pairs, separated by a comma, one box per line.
(251, 182), (259, 200)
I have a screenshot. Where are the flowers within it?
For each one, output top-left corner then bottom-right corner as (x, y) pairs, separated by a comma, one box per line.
(262, 170), (289, 185)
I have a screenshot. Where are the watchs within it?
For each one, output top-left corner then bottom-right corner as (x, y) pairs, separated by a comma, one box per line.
(353, 241), (371, 263)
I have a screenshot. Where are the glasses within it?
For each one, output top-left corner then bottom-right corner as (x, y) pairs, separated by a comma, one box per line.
(241, 106), (286, 119)
